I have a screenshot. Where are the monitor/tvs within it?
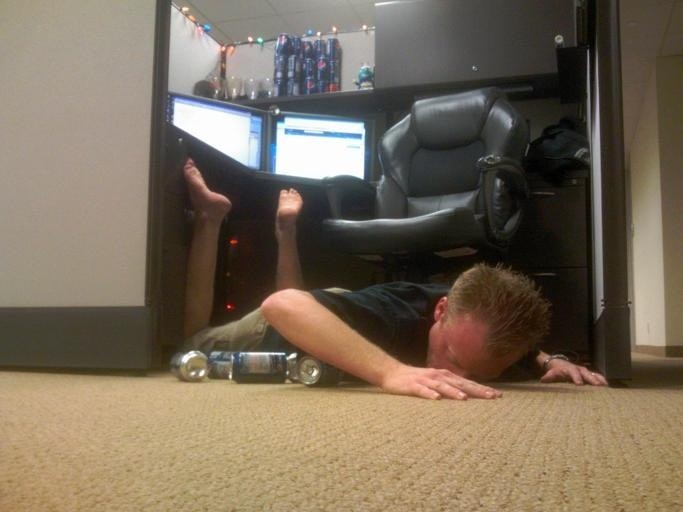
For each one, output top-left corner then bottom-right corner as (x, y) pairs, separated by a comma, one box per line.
(269, 109), (376, 189)
(166, 90), (267, 179)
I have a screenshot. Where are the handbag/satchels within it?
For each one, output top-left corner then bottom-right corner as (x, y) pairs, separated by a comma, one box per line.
(528, 124), (588, 176)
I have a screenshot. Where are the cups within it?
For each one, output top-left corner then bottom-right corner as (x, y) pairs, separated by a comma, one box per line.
(225, 75), (242, 99)
(261, 78), (274, 97)
(208, 77), (225, 99)
(244, 77), (260, 100)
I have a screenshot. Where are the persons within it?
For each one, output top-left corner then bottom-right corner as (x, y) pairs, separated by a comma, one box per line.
(180, 160), (609, 400)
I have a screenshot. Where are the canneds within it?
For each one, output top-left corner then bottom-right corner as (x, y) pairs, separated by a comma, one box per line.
(272, 33), (341, 97)
(170, 350), (338, 386)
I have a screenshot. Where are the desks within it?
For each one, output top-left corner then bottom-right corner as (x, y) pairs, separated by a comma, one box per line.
(162, 121), (378, 353)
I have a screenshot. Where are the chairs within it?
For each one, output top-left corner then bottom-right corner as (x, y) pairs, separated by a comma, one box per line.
(319, 86), (532, 285)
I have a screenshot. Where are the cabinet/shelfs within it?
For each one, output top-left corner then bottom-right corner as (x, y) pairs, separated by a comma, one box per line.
(467, 170), (593, 372)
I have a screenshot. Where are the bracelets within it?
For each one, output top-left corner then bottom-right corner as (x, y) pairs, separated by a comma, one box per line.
(543, 353), (568, 371)
